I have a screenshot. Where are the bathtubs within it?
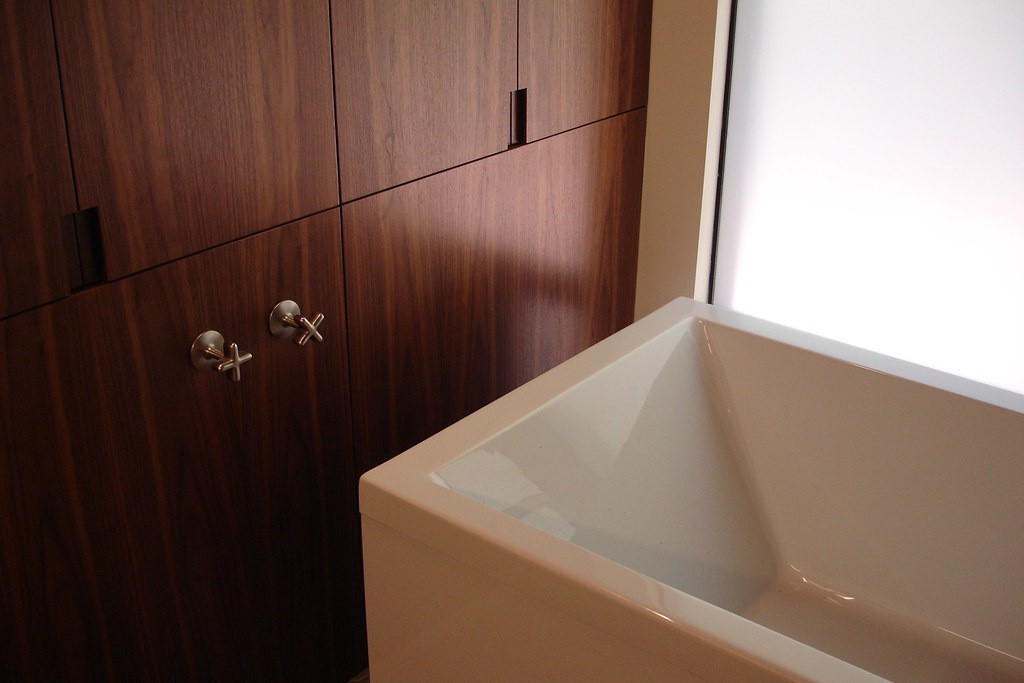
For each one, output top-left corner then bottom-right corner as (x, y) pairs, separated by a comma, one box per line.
(359, 296), (1024, 683)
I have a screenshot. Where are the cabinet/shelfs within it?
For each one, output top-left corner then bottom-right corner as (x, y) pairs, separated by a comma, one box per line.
(0, 0), (653, 683)
